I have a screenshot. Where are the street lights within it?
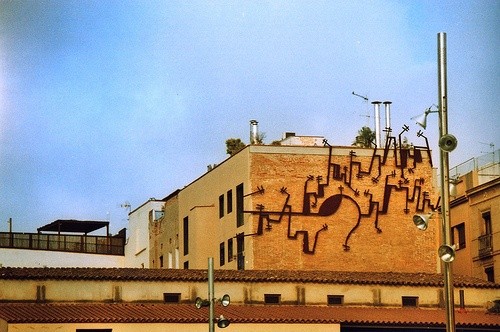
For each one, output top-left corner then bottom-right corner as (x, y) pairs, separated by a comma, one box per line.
(411, 30), (459, 332)
(195, 257), (231, 332)
